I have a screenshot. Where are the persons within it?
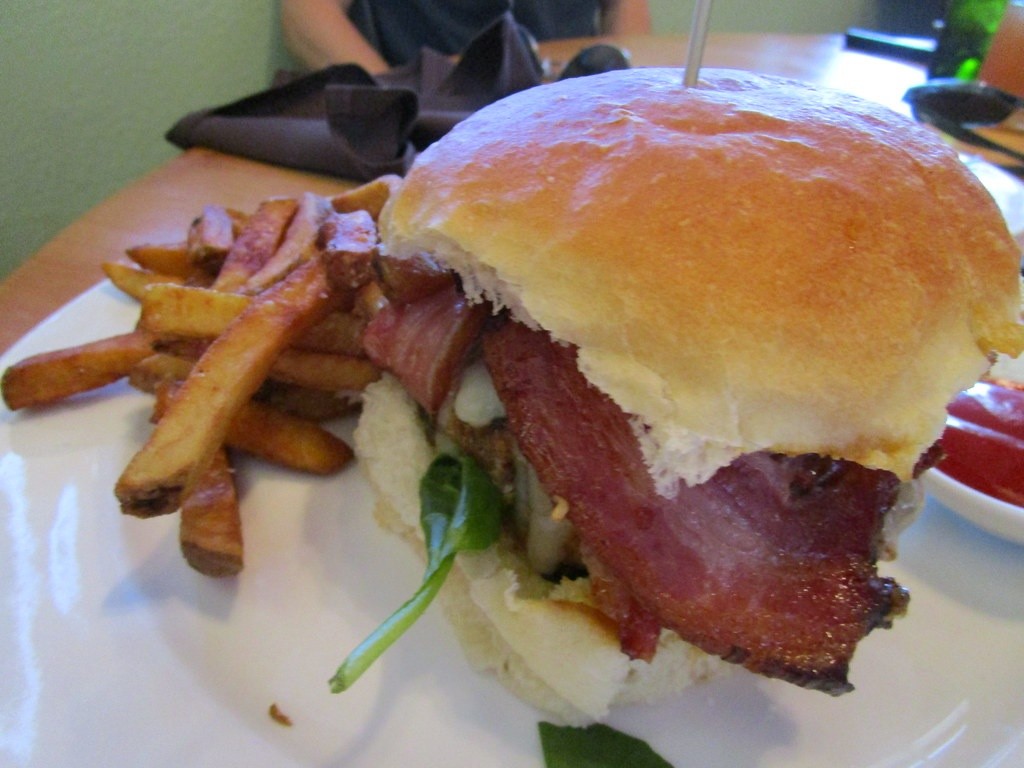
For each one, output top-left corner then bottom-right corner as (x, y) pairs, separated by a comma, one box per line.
(265, 0), (653, 89)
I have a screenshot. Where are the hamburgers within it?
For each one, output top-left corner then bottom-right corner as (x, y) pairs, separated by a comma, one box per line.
(325, 68), (1023, 768)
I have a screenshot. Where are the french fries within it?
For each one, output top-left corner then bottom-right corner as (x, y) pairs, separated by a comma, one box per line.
(0, 174), (399, 575)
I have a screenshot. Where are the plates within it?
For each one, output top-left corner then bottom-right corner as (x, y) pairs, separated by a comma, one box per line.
(913, 339), (1023, 549)
(0, 267), (1023, 766)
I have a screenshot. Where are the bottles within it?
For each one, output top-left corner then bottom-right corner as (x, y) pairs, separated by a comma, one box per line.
(925, 0), (1007, 79)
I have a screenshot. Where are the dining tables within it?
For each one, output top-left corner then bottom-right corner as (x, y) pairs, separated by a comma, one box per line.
(0, 27), (1022, 367)
